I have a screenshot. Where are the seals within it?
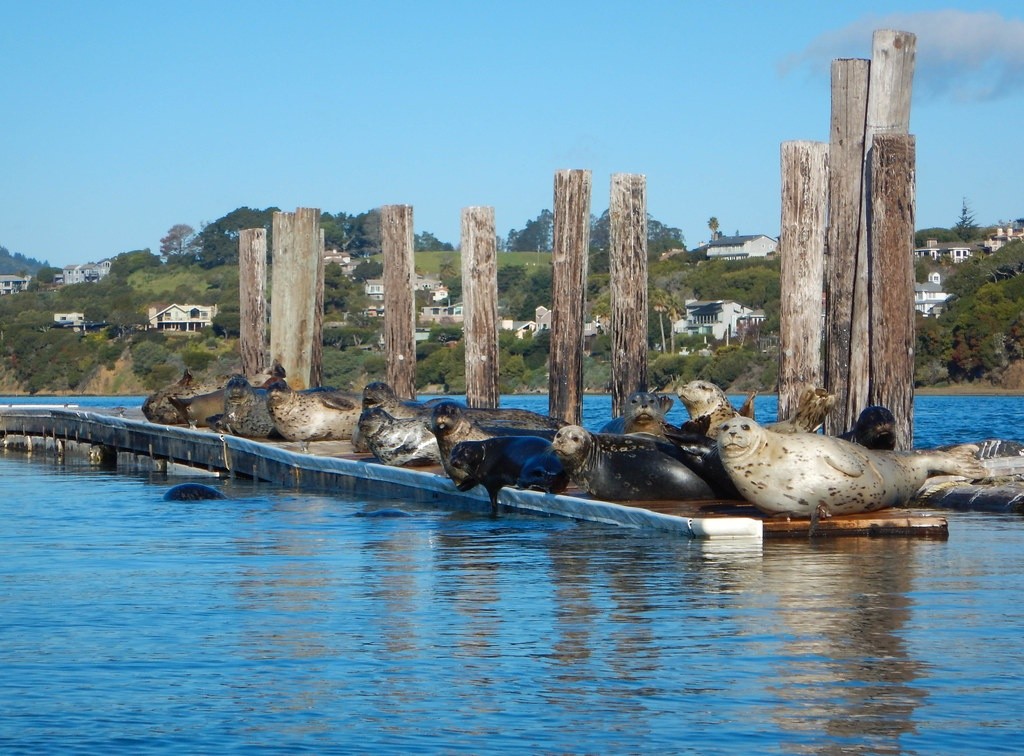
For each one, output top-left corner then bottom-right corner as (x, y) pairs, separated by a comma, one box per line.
(353, 379), (990, 537)
(162, 482), (228, 502)
(141, 359), (362, 442)
